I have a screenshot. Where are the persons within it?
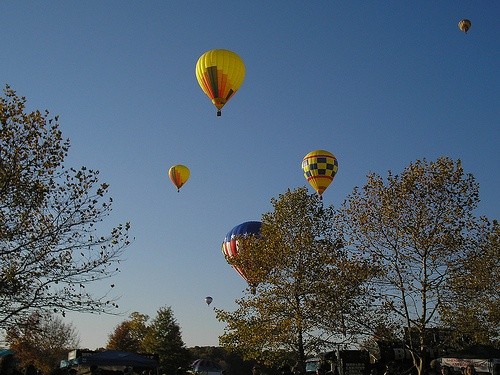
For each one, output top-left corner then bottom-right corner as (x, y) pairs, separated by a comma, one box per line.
(0, 348), (500, 375)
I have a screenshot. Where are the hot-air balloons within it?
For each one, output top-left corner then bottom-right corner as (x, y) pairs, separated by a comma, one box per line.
(205, 296), (213, 307)
(169, 165), (191, 193)
(221, 219), (280, 295)
(195, 48), (246, 117)
(458, 18), (471, 35)
(301, 150), (340, 200)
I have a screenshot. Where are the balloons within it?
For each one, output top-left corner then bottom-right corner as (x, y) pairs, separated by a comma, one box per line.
(170, 164), (190, 193)
(222, 221), (270, 295)
(194, 48), (247, 117)
(299, 149), (339, 201)
(205, 296), (213, 304)
(457, 17), (473, 33)
